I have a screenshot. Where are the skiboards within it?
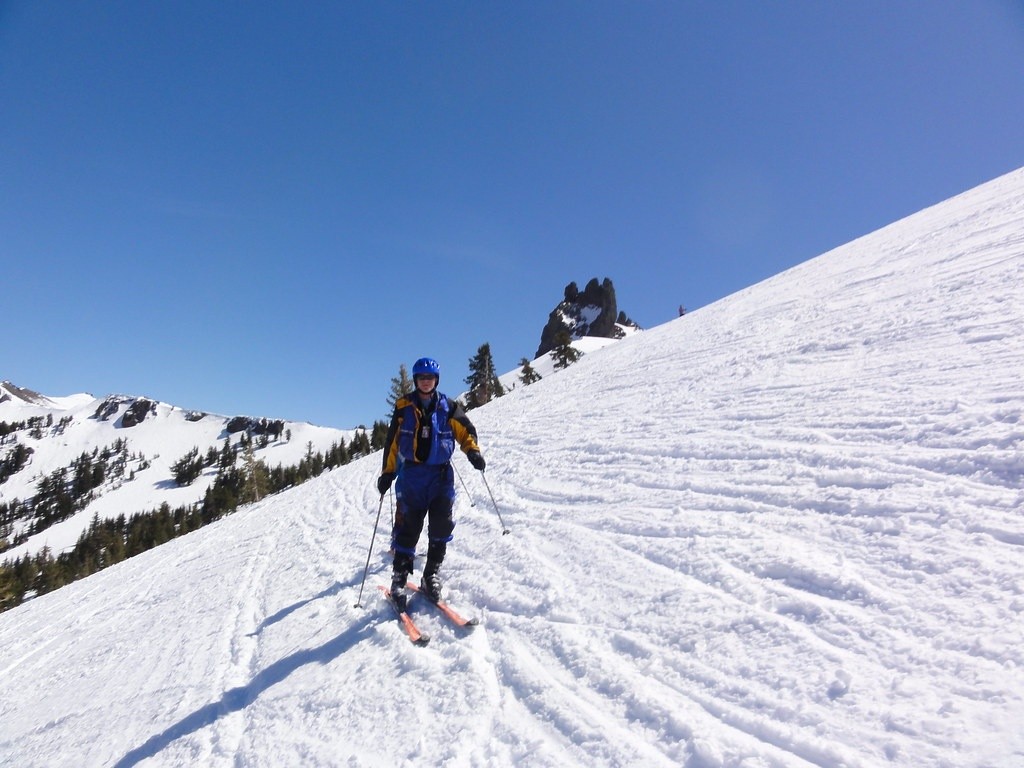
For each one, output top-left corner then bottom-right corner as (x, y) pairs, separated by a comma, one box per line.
(377, 579), (480, 646)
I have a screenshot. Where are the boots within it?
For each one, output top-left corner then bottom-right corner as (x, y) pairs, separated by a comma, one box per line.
(421, 558), (443, 600)
(389, 568), (415, 602)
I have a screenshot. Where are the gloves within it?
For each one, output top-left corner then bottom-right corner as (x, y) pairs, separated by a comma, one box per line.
(468, 451), (486, 470)
(377, 473), (397, 495)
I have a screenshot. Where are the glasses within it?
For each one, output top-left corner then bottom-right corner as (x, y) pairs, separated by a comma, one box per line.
(417, 374), (435, 380)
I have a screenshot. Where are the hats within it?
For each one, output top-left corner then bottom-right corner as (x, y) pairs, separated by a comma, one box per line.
(412, 358), (440, 386)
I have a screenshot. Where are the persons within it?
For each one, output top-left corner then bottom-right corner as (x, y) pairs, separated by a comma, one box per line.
(679, 305), (686, 316)
(378, 358), (486, 610)
